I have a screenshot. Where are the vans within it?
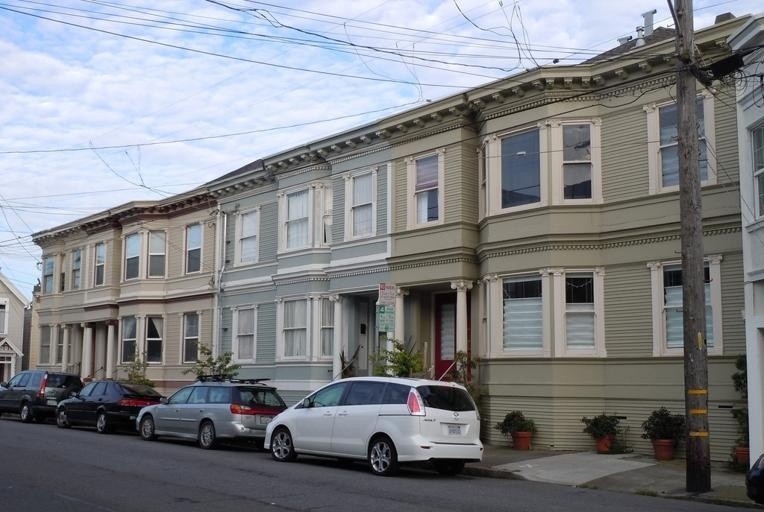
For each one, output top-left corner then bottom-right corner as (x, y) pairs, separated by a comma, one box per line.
(263, 362), (485, 476)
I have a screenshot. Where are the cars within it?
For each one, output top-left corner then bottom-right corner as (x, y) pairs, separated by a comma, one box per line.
(55, 381), (167, 434)
(745, 454), (763, 506)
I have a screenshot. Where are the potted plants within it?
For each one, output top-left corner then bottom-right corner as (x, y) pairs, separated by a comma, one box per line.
(580, 410), (620, 455)
(493, 410), (537, 449)
(640, 403), (683, 460)
(729, 351), (753, 469)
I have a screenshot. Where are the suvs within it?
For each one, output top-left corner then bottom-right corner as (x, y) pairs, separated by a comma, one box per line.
(134, 374), (288, 451)
(0, 369), (84, 426)
